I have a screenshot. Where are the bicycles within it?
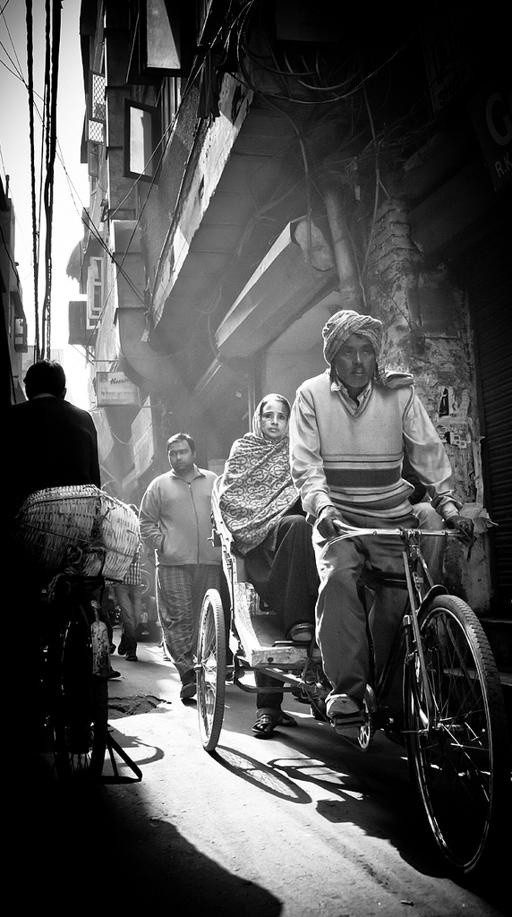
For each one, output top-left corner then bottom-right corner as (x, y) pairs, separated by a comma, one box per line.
(9, 532), (147, 790)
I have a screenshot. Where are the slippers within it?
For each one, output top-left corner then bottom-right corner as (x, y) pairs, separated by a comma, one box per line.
(251, 709), (297, 738)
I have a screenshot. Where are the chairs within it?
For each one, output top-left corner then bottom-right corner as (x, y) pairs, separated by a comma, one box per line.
(210, 473), (322, 668)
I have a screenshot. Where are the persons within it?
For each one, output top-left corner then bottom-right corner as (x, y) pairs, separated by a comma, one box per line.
(0, 357), (105, 758)
(286, 308), (477, 743)
(136, 431), (220, 699)
(219, 390), (323, 646)
(110, 501), (143, 663)
(403, 469), (452, 588)
(246, 670), (296, 740)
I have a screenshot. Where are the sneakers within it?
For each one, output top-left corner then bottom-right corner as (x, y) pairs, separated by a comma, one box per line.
(118, 633), (138, 661)
(178, 679), (198, 700)
(324, 694), (362, 740)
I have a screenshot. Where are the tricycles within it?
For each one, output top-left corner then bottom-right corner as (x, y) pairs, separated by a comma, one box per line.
(184, 501), (506, 881)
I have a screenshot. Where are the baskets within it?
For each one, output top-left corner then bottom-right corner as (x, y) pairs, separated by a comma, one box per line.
(15, 483), (139, 583)
